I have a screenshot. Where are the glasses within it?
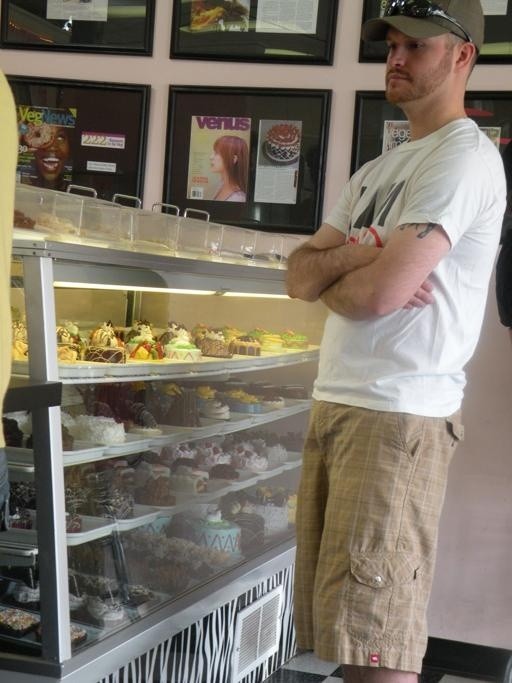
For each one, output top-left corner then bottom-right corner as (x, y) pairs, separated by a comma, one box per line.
(386, 0), (473, 42)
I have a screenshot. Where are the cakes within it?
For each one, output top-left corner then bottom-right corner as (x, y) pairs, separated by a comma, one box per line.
(11, 321), (311, 365)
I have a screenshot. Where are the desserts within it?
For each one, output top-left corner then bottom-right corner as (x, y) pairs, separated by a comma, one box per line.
(0, 379), (310, 647)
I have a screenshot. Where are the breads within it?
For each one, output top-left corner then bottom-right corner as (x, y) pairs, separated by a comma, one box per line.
(36, 212), (70, 228)
(13, 209), (34, 227)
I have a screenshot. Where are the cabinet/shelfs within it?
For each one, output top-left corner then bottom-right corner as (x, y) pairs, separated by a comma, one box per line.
(0, 235), (330, 683)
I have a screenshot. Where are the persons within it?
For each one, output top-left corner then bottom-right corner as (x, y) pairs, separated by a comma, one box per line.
(22, 106), (78, 191)
(285, 1), (510, 683)
(210, 135), (250, 202)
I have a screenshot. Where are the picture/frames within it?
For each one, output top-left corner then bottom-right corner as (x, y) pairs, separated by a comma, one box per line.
(358, 1), (512, 67)
(352, 90), (511, 242)
(1, 0), (158, 56)
(161, 80), (334, 236)
(3, 73), (153, 209)
(169, 1), (341, 67)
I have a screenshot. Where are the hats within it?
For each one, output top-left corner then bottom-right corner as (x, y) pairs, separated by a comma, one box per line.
(364, 0), (485, 53)
